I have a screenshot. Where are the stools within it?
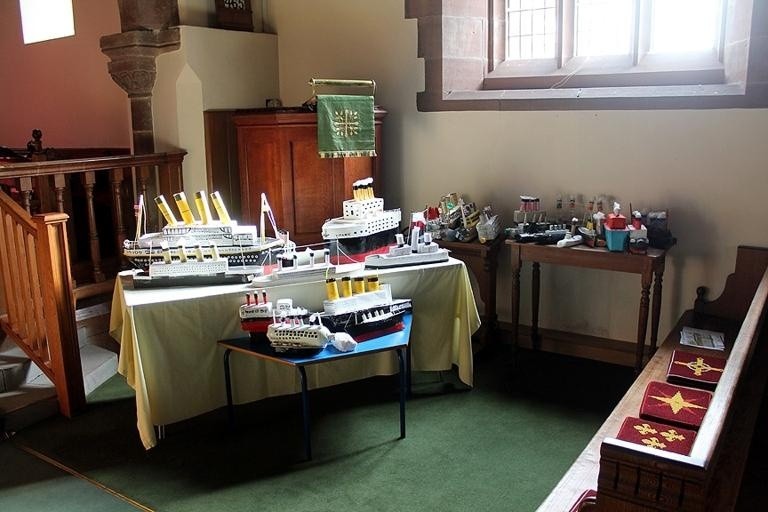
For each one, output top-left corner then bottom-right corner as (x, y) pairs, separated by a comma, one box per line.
(216, 312), (413, 459)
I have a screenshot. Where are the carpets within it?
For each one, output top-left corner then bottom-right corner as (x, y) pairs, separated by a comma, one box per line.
(0, 335), (640, 511)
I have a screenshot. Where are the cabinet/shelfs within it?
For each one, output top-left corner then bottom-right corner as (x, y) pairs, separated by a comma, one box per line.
(202, 106), (387, 250)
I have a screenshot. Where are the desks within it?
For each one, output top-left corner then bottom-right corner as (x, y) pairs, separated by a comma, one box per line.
(407, 230), (504, 351)
(109, 256), (482, 450)
(505, 238), (666, 373)
